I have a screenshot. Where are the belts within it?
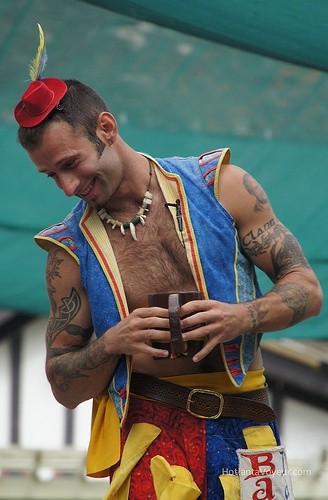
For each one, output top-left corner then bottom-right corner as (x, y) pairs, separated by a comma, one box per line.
(131, 374), (277, 424)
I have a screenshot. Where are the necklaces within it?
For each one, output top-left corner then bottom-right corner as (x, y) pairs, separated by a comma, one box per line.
(97, 159), (153, 241)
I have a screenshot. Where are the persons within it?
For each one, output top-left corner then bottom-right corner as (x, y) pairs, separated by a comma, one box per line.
(18, 80), (323, 500)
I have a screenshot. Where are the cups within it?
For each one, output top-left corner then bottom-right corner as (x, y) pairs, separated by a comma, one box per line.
(146, 290), (203, 362)
